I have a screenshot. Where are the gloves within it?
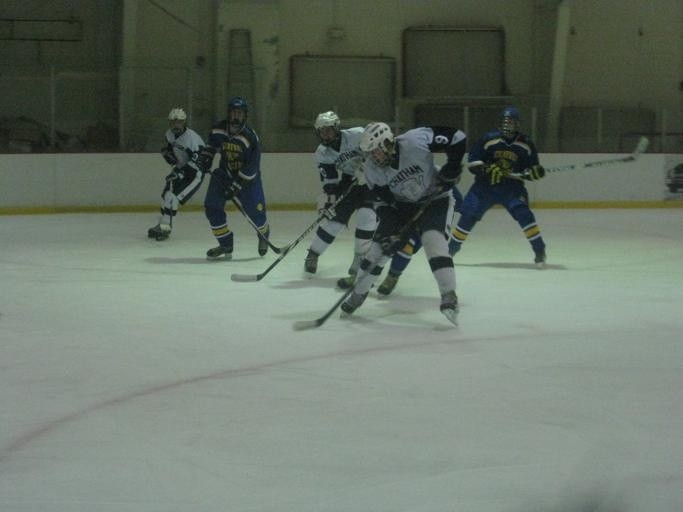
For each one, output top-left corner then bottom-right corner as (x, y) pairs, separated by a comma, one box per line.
(523, 165), (544, 180)
(197, 155), (211, 172)
(165, 167), (184, 186)
(351, 163), (365, 186)
(480, 160), (503, 185)
(433, 164), (459, 192)
(380, 232), (402, 257)
(224, 185), (237, 200)
(318, 203), (336, 220)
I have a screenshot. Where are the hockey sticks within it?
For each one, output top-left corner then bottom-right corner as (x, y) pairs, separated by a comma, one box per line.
(294, 184), (445, 331)
(230, 179), (357, 282)
(186, 147), (291, 254)
(506, 135), (650, 180)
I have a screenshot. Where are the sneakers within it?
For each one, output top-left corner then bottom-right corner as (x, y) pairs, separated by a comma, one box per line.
(154, 230), (169, 240)
(337, 275), (353, 289)
(304, 248), (319, 274)
(148, 224), (159, 235)
(439, 290), (457, 311)
(341, 291), (368, 313)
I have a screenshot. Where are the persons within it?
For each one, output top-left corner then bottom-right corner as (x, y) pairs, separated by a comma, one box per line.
(200, 96), (270, 259)
(336, 165), (464, 297)
(339, 121), (468, 316)
(303, 110), (378, 278)
(447, 106), (547, 266)
(147, 108), (206, 241)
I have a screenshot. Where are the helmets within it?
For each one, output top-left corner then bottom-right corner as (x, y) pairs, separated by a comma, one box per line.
(314, 111), (340, 128)
(228, 97), (246, 111)
(359, 121), (393, 168)
(167, 108), (186, 121)
(494, 108), (518, 135)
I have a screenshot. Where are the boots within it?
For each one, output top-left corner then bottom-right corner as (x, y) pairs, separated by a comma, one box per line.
(257, 223), (269, 255)
(207, 231), (233, 256)
(534, 248), (544, 264)
(377, 273), (401, 295)
